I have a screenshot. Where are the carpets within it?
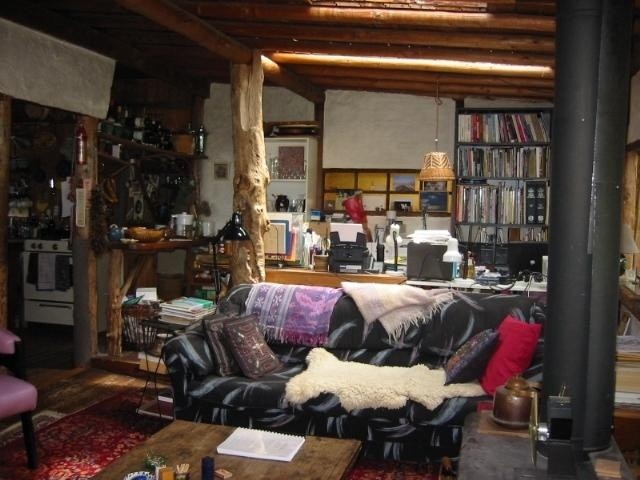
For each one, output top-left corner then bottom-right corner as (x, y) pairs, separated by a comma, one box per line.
(1, 389), (456, 480)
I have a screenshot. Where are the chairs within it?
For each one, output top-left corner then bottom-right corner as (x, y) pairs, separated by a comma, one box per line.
(0, 328), (38, 470)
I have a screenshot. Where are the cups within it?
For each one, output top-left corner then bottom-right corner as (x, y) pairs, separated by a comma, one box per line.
(203, 222), (215, 237)
(265, 153), (308, 179)
(314, 254), (331, 272)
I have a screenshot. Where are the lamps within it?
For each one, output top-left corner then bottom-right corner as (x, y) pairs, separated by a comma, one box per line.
(386, 210), (396, 229)
(618, 223), (640, 275)
(417, 238), (476, 281)
(419, 79), (456, 182)
(383, 222), (405, 278)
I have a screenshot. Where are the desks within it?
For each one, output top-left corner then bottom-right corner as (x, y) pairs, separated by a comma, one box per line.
(265, 260), (546, 304)
(86, 419), (363, 480)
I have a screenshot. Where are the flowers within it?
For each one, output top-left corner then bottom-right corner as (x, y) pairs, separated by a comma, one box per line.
(338, 188), (373, 240)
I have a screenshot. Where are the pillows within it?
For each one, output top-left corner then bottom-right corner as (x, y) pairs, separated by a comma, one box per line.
(478, 314), (543, 397)
(445, 327), (499, 384)
(202, 311), (242, 377)
(222, 313), (281, 378)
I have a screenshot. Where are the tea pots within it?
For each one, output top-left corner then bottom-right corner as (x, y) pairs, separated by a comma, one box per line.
(490, 373), (544, 428)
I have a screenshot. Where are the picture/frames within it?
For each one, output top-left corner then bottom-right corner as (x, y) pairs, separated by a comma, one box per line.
(390, 199), (412, 214)
(419, 192), (447, 213)
(325, 199), (335, 212)
(424, 179), (447, 191)
(391, 173), (415, 192)
(507, 227), (520, 244)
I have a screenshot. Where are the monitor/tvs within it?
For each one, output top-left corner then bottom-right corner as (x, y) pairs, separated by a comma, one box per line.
(507, 241), (549, 279)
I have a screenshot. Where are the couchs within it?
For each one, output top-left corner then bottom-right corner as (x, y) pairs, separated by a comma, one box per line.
(161, 282), (502, 475)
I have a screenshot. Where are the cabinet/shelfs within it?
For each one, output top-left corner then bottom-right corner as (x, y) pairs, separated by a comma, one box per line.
(265, 120), (318, 230)
(93, 237), (233, 374)
(320, 167), (454, 217)
(450, 109), (553, 273)
(73, 118), (207, 378)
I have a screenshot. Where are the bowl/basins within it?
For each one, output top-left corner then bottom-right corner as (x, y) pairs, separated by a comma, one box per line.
(123, 470), (156, 480)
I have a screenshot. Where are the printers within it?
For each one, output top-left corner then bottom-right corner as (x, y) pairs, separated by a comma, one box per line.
(328, 231), (371, 273)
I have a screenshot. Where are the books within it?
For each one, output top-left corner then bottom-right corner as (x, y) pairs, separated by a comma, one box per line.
(215, 426), (305, 463)
(155, 295), (215, 326)
(137, 391), (173, 420)
(455, 111), (551, 244)
(263, 211), (322, 268)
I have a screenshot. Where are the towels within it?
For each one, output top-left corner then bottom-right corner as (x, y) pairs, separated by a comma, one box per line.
(25, 252), (70, 291)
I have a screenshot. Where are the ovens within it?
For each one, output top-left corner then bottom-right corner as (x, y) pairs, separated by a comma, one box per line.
(21, 250), (76, 327)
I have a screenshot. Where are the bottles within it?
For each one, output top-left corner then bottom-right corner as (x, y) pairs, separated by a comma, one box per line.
(8, 214), (71, 239)
(270, 193), (305, 213)
(155, 466), (190, 480)
(101, 101), (208, 156)
(201, 455), (215, 480)
(106, 210), (202, 244)
